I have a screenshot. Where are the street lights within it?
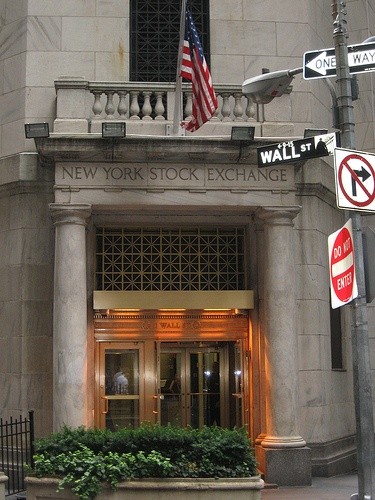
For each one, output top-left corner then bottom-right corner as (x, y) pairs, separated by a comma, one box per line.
(239, 58), (375, 499)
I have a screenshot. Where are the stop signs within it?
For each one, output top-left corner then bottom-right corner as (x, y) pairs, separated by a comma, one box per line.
(327, 217), (360, 310)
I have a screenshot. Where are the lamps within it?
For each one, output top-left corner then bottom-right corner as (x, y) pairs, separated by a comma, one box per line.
(102, 122), (128, 138)
(231, 309), (241, 315)
(231, 126), (257, 141)
(25, 122), (50, 138)
(303, 128), (328, 138)
(99, 309), (110, 315)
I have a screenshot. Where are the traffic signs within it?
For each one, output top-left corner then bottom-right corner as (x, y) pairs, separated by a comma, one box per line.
(302, 40), (375, 81)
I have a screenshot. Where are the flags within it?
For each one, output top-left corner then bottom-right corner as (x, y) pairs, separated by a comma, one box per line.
(179, 4), (218, 133)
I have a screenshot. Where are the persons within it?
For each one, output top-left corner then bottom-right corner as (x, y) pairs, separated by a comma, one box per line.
(111, 366), (128, 395)
(164, 358), (176, 394)
(206, 362), (220, 426)
